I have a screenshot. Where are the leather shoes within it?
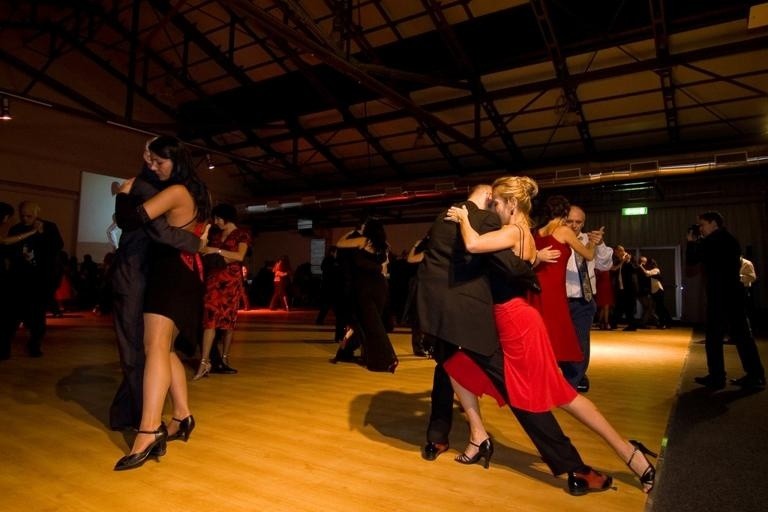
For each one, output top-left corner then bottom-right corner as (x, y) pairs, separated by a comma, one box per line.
(731, 373), (764, 386)
(596, 322), (671, 331)
(694, 374), (725, 389)
(568, 469), (612, 495)
(424, 441), (449, 460)
(577, 375), (589, 391)
(209, 364), (238, 374)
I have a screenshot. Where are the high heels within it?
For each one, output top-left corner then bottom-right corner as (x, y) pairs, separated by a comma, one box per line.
(455, 438), (493, 469)
(223, 355), (232, 367)
(114, 420), (168, 470)
(166, 415), (195, 441)
(0, 347), (11, 359)
(193, 359), (211, 381)
(387, 358), (398, 374)
(330, 349), (347, 362)
(628, 440), (657, 493)
(25, 343), (43, 357)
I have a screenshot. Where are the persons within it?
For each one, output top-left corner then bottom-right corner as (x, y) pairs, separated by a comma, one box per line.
(182, 202), (311, 381)
(725, 255), (759, 345)
(1, 201), (101, 362)
(97, 134), (211, 469)
(409, 178), (673, 496)
(315, 218), (416, 373)
(685, 211), (765, 390)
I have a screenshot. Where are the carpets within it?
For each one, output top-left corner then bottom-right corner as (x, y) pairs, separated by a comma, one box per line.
(644, 322), (768, 512)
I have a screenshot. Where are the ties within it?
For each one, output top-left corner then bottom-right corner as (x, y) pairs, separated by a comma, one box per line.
(574, 239), (593, 303)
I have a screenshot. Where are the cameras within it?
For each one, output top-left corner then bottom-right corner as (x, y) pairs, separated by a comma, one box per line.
(688, 223), (701, 237)
